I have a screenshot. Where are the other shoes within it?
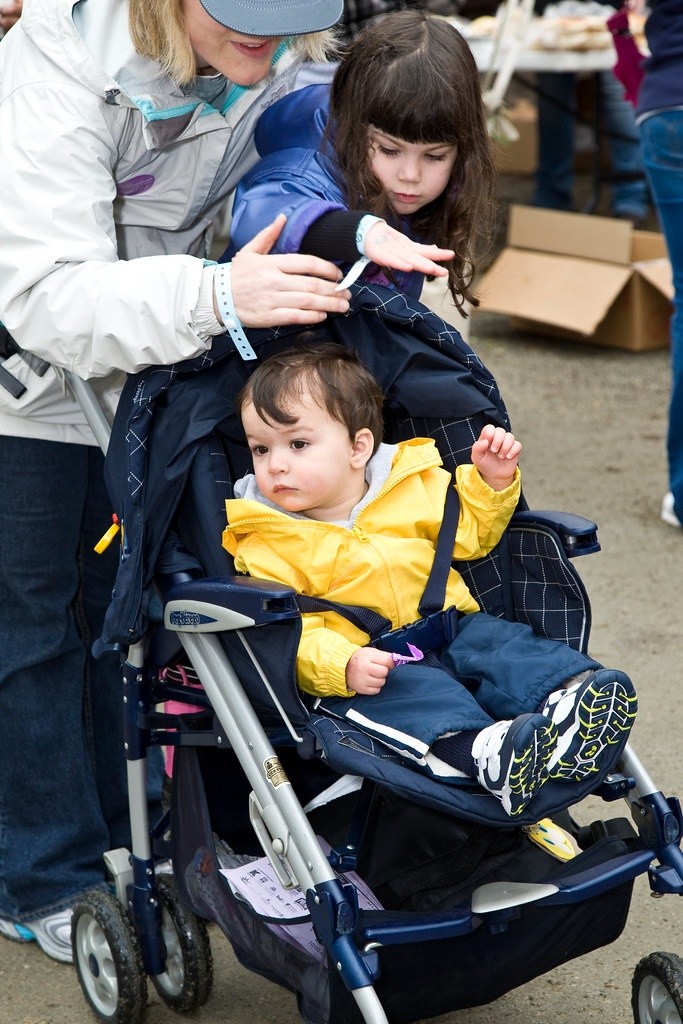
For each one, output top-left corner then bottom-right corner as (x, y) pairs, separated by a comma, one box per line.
(660, 493), (681, 527)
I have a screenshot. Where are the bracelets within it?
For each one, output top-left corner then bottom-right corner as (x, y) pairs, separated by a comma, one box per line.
(213, 262), (258, 361)
(335, 215), (386, 291)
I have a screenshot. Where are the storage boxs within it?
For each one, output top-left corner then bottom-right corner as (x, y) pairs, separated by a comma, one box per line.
(473, 203), (676, 350)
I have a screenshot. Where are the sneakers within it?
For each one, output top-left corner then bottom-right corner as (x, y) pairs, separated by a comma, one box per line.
(470, 711), (558, 818)
(540, 669), (638, 785)
(0, 907), (75, 963)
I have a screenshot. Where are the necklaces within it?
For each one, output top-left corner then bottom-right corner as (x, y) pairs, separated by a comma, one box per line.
(196, 66), (213, 77)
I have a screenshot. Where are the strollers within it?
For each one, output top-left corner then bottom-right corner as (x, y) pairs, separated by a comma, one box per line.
(65, 277), (683, 1024)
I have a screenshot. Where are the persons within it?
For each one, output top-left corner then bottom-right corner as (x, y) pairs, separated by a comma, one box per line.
(218, 9), (503, 319)
(0, 0), (349, 964)
(219, 341), (640, 820)
(527, 0), (683, 528)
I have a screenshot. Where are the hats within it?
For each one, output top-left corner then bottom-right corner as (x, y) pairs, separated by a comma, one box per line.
(201, 0), (344, 37)
